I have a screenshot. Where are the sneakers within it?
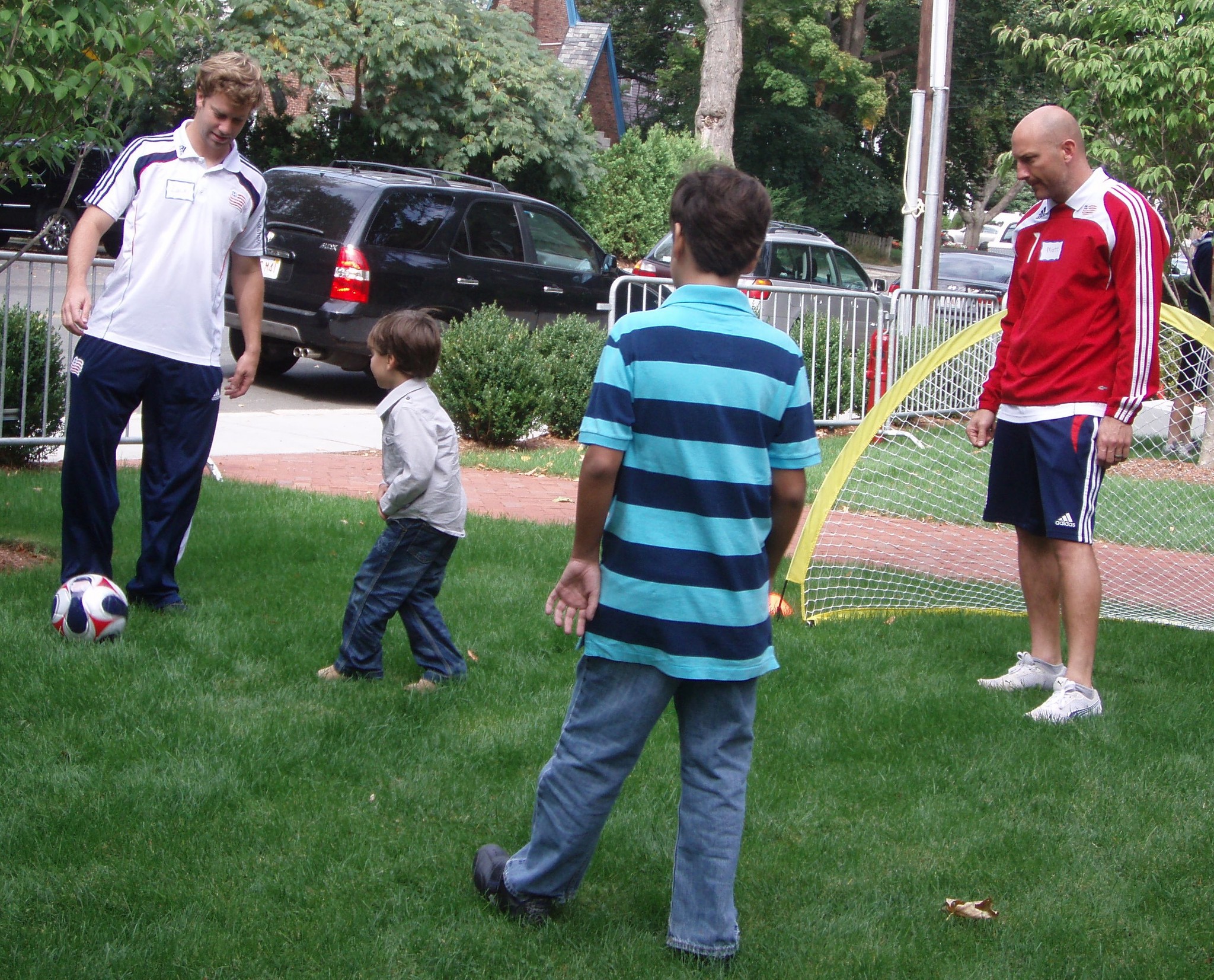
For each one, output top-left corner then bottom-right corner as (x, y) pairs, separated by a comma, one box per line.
(472, 843), (560, 926)
(977, 651), (1068, 692)
(1022, 676), (1102, 723)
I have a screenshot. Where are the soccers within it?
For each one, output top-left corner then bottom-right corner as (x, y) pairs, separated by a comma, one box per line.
(48, 572), (128, 643)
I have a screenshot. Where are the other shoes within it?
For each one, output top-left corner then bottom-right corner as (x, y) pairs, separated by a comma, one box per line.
(1164, 440), (1193, 460)
(404, 677), (436, 693)
(158, 599), (188, 613)
(1185, 439), (1201, 450)
(318, 666), (341, 681)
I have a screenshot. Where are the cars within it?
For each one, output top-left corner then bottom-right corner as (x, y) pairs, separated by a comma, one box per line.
(882, 181), (1189, 335)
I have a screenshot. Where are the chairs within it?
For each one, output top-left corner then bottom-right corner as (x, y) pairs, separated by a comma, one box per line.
(758, 253), (784, 277)
(794, 254), (819, 280)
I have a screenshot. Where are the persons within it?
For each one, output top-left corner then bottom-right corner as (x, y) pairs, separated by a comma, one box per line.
(471, 163), (820, 967)
(1163, 230), (1214, 458)
(60, 52), (268, 610)
(966, 105), (1171, 719)
(318, 310), (471, 690)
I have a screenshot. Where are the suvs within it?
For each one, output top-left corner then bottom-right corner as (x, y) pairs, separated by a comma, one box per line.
(0, 134), (124, 258)
(620, 220), (899, 405)
(224, 160), (667, 395)
